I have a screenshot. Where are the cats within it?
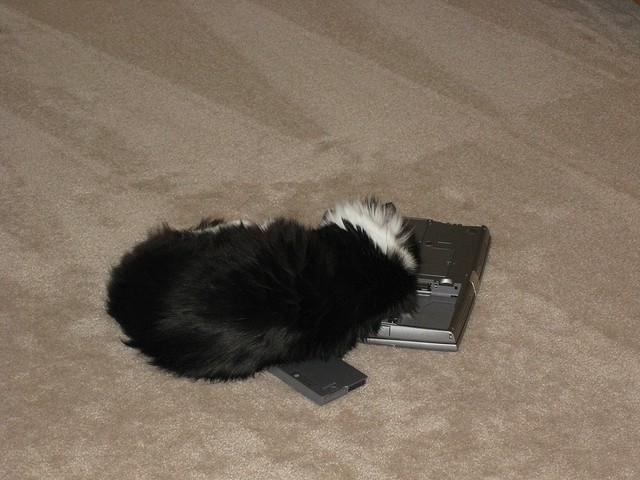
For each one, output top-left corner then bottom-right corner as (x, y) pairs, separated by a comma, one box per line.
(103, 195), (422, 384)
